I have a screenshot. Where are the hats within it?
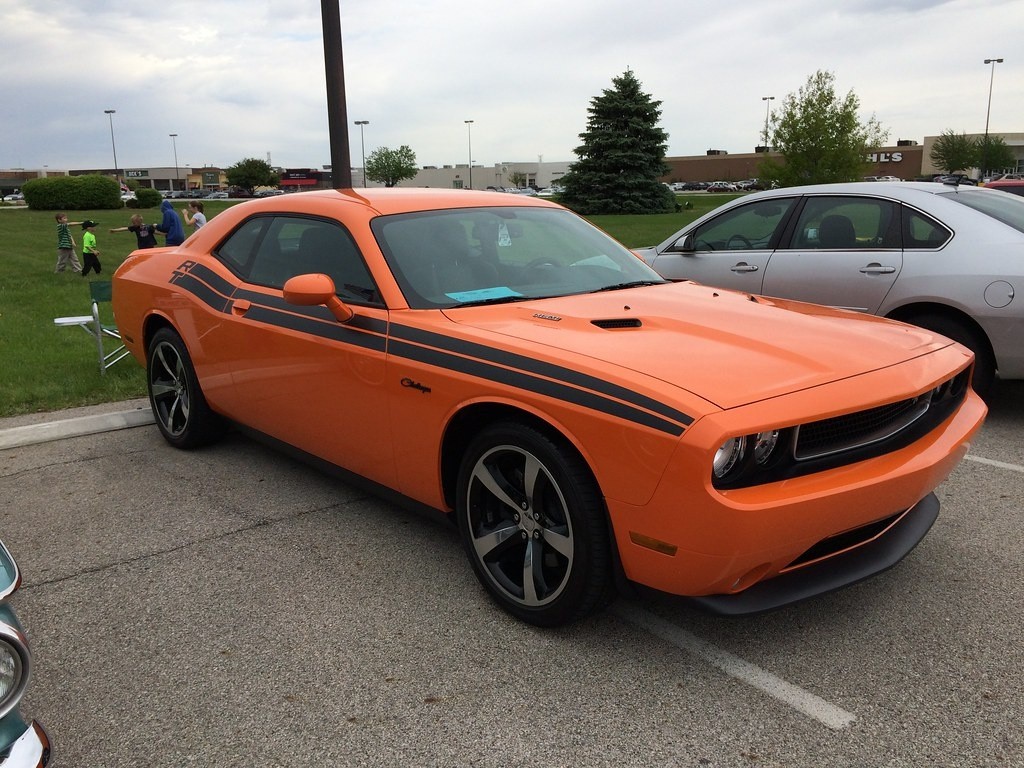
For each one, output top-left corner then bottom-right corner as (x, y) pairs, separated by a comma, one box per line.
(81, 220), (99, 230)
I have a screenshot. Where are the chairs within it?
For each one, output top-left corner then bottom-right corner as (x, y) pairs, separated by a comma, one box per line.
(412, 219), (499, 297)
(815, 216), (857, 248)
(282, 227), (376, 301)
(54, 280), (131, 375)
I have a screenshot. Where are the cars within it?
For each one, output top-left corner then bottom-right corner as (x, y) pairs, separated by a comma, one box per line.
(863, 174), (1024, 189)
(121, 190), (285, 200)
(3, 193), (25, 200)
(566, 180), (1024, 401)
(506, 186), (566, 195)
(662, 181), (780, 193)
(110, 188), (990, 633)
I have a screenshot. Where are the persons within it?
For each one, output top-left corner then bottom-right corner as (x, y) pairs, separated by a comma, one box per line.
(109, 214), (167, 250)
(153, 200), (184, 247)
(54, 213), (83, 272)
(181, 200), (206, 232)
(82, 221), (103, 276)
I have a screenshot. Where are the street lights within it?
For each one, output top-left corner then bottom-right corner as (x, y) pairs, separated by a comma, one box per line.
(354, 121), (369, 188)
(465, 120), (473, 189)
(984, 59), (1003, 139)
(169, 134), (181, 188)
(105, 109), (120, 183)
(762, 96), (775, 151)
